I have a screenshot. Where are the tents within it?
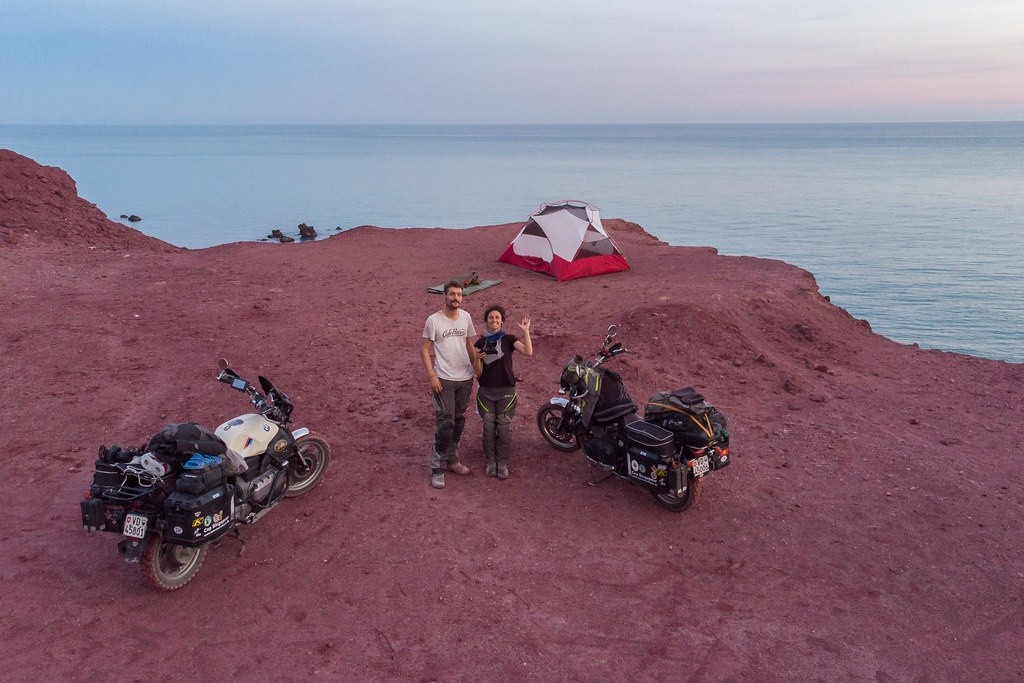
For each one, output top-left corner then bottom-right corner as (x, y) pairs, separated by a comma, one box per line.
(496, 200), (631, 282)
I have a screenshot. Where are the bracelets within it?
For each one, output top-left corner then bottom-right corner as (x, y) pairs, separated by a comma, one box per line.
(429, 374), (435, 378)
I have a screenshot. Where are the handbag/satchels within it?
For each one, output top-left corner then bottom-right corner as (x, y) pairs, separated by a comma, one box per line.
(80, 497), (105, 531)
(90, 422), (249, 504)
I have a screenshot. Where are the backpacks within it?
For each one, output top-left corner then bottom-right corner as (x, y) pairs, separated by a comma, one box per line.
(644, 388), (727, 432)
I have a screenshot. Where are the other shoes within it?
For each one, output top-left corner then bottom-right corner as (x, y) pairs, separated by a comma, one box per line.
(447, 462), (470, 475)
(498, 465), (508, 479)
(432, 473), (445, 488)
(486, 463), (497, 477)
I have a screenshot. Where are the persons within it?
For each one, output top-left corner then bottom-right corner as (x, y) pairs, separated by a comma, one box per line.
(421, 281), (476, 488)
(473, 305), (532, 478)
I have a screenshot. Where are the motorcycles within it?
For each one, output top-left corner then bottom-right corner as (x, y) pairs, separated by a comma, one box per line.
(537, 325), (731, 513)
(81, 357), (332, 591)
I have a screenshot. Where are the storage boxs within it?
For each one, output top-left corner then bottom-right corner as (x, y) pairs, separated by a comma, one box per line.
(160, 487), (236, 545)
(82, 459), (149, 534)
(708, 442), (731, 469)
(628, 448), (669, 491)
